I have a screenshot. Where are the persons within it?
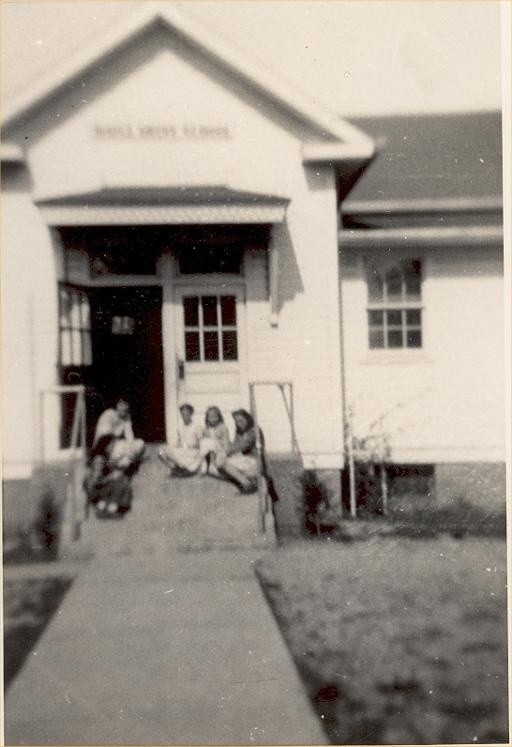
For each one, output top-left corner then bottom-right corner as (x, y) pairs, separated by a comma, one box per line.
(224, 410), (265, 492)
(83, 434), (132, 518)
(200, 407), (230, 477)
(92, 396), (145, 469)
(158, 405), (202, 475)
